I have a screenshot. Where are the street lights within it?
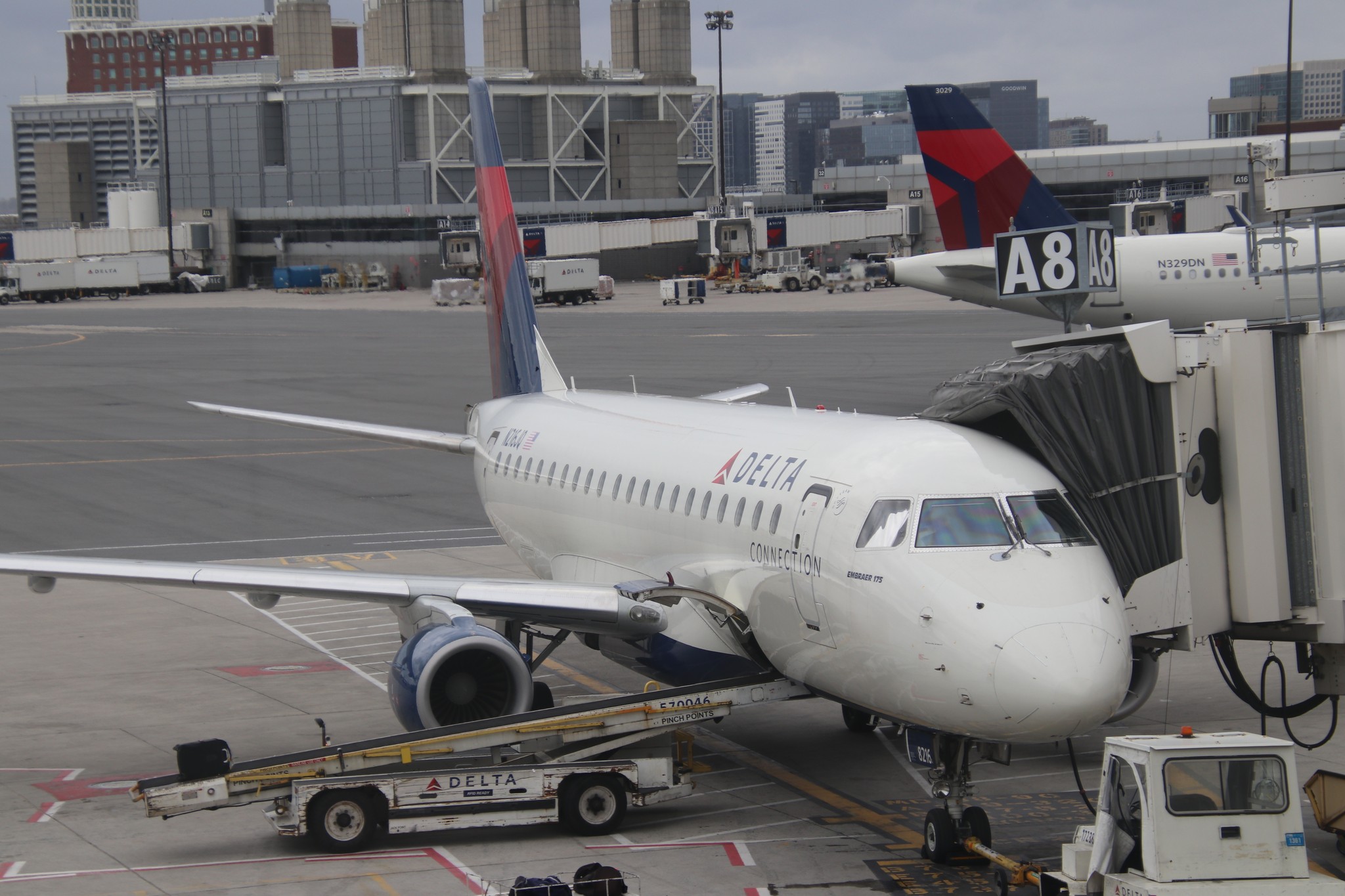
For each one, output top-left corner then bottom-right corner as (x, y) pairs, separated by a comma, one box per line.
(704, 9), (735, 219)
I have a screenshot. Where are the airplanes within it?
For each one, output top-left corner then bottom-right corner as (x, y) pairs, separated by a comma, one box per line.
(1, 74), (1345, 867)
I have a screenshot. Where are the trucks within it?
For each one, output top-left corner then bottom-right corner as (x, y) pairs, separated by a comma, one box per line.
(0, 259), (140, 305)
(525, 259), (600, 306)
(762, 264), (822, 293)
